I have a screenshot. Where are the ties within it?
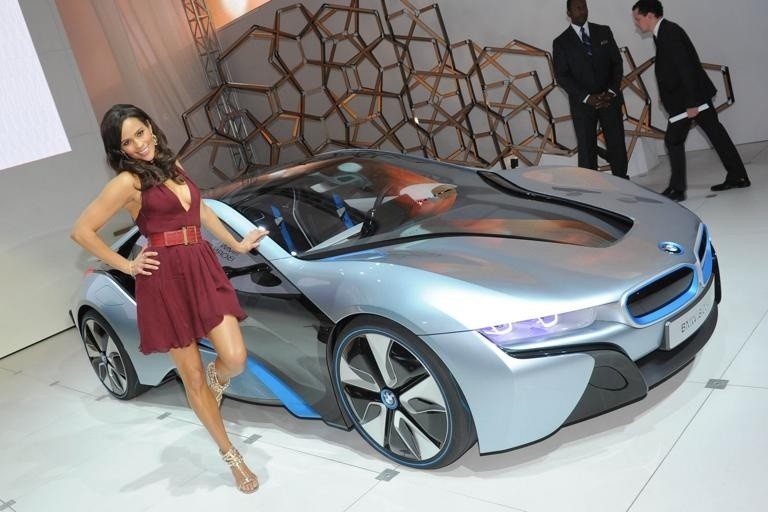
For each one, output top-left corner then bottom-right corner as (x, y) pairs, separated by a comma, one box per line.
(580, 26), (593, 57)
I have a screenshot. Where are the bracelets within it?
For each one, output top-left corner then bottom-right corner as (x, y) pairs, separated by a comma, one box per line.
(130, 259), (136, 279)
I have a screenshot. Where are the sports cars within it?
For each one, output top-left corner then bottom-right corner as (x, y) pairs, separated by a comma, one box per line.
(68, 148), (721, 469)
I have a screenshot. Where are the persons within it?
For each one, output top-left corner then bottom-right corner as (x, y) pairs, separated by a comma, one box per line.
(631, 0), (751, 203)
(69, 104), (273, 494)
(552, 0), (630, 183)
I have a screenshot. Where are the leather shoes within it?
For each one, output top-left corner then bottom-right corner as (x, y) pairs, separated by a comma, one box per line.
(661, 186), (686, 202)
(711, 173), (751, 191)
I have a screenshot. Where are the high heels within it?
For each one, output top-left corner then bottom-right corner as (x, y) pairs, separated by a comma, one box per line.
(220, 442), (260, 495)
(206, 360), (230, 407)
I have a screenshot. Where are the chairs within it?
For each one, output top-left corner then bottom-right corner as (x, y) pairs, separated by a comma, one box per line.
(291, 179), (348, 252)
(238, 196), (303, 257)
